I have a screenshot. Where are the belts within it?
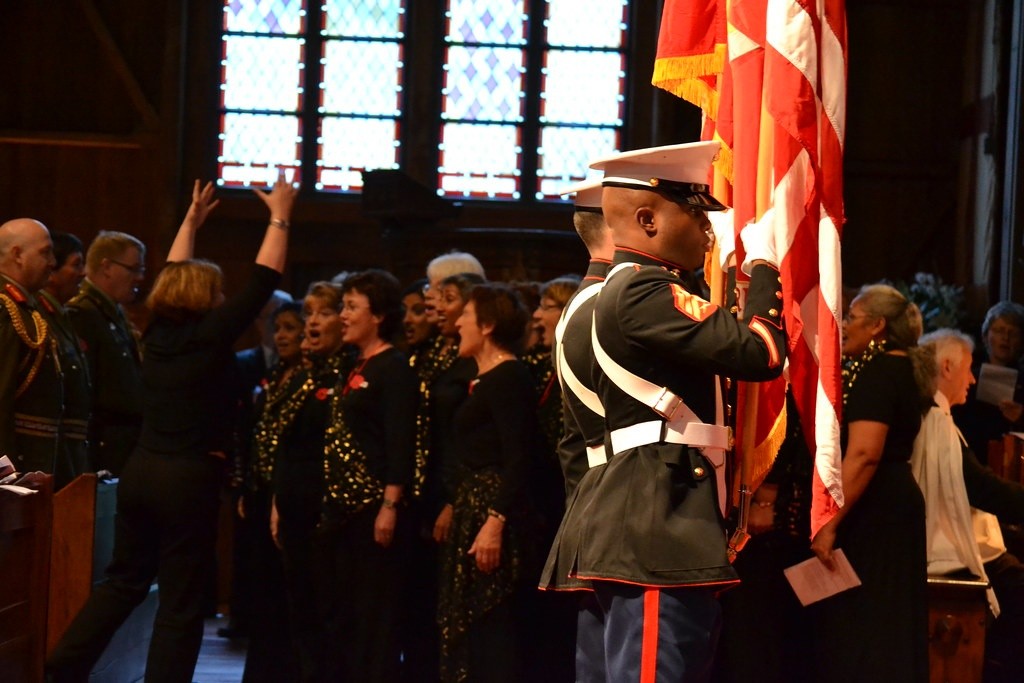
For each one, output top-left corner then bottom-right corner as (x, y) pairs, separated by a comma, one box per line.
(13, 412), (58, 437)
(608, 417), (735, 456)
(60, 418), (89, 439)
(585, 443), (608, 468)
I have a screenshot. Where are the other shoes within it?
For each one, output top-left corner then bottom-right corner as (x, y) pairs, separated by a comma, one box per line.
(216, 622), (243, 639)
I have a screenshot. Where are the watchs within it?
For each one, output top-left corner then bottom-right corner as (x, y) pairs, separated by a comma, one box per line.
(381, 498), (397, 509)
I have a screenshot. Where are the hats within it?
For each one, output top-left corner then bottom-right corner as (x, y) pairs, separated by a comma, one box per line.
(571, 174), (604, 214)
(593, 140), (728, 211)
(427, 251), (486, 286)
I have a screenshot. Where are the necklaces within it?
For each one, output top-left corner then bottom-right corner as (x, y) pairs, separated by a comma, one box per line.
(836, 339), (900, 410)
(477, 350), (510, 377)
(338, 340), (389, 400)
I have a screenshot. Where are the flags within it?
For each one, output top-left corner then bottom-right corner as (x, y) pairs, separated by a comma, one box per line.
(653, 0), (846, 542)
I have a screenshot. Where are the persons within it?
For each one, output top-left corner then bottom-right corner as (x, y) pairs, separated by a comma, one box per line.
(0, 216), (144, 594)
(41, 177), (302, 683)
(722, 279), (1024, 683)
(556, 137), (789, 683)
(215, 251), (580, 683)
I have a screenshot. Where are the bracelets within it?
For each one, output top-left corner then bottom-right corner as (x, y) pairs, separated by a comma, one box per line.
(751, 498), (777, 508)
(269, 216), (291, 230)
(488, 509), (507, 522)
(447, 504), (453, 508)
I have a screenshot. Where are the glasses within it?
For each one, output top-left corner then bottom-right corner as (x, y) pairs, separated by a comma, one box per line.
(539, 303), (563, 311)
(845, 311), (874, 325)
(111, 259), (146, 278)
